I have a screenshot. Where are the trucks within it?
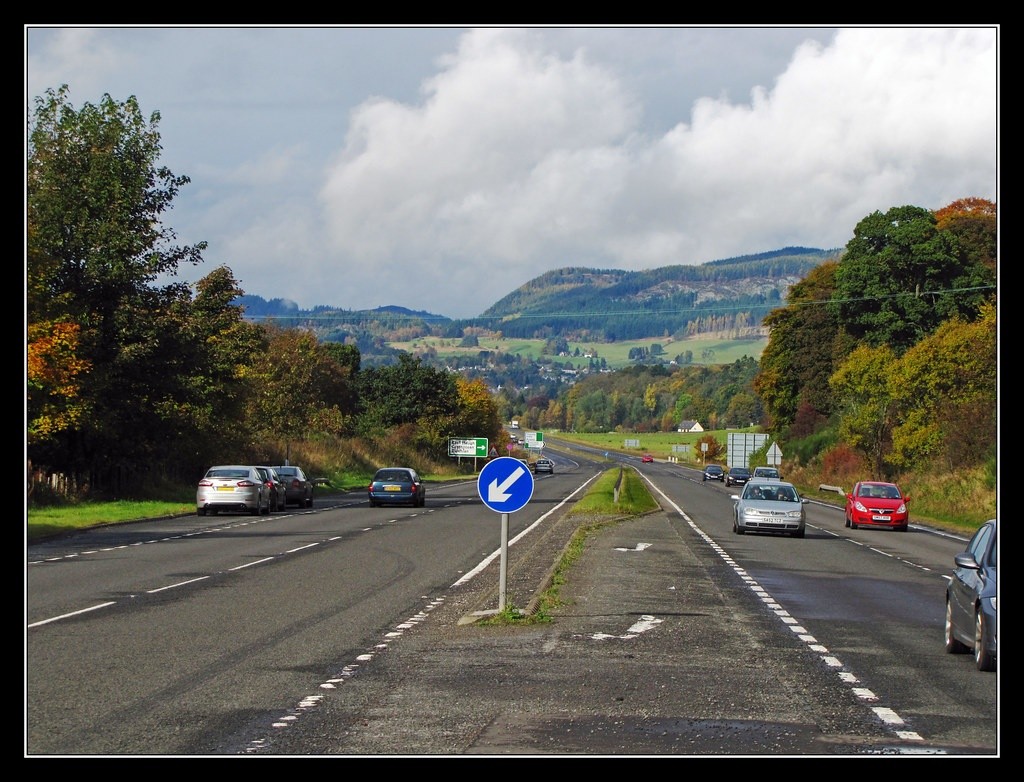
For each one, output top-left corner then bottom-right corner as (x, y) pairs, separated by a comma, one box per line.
(511, 420), (520, 429)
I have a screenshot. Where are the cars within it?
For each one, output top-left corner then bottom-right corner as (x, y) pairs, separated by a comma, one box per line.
(509, 433), (525, 446)
(642, 453), (653, 463)
(520, 459), (554, 474)
(944, 519), (997, 672)
(750, 467), (784, 482)
(725, 468), (752, 487)
(844, 480), (911, 532)
(369, 467), (426, 508)
(730, 480), (809, 538)
(703, 465), (725, 483)
(196, 466), (287, 516)
(271, 466), (313, 508)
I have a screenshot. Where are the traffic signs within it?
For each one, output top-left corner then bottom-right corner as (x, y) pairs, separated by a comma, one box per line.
(448, 438), (489, 458)
